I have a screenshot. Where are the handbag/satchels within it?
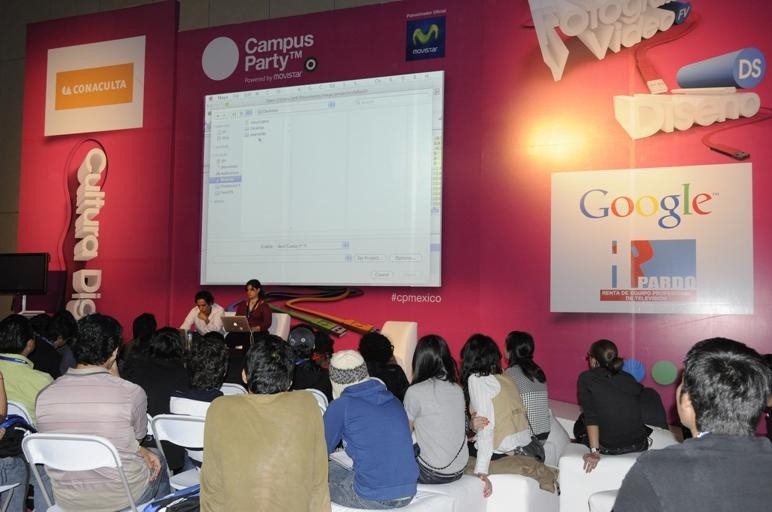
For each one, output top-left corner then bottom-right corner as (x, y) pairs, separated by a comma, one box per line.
(514, 438), (545, 463)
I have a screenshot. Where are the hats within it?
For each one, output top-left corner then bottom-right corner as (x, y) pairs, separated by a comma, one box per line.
(329, 349), (370, 400)
(289, 327), (316, 354)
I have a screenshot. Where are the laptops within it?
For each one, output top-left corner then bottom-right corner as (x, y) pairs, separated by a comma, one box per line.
(220, 315), (251, 333)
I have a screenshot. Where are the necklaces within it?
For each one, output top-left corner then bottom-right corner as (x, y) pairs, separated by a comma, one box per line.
(247, 297), (260, 320)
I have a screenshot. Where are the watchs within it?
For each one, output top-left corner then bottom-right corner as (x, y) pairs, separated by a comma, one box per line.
(590, 448), (600, 453)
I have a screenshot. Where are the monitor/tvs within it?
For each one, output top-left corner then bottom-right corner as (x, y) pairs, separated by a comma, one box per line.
(0, 252), (47, 295)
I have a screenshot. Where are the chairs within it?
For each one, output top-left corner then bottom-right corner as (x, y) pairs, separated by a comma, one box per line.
(379, 320), (417, 385)
(306, 387), (328, 416)
(146, 413), (153, 436)
(7, 400), (34, 426)
(21, 432), (154, 512)
(170, 396), (210, 414)
(219, 382), (248, 396)
(223, 311), (237, 338)
(150, 415), (206, 489)
(268, 312), (291, 342)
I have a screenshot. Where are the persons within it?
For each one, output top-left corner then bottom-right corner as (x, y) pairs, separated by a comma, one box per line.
(179, 291), (225, 342)
(1, 309), (417, 512)
(573, 339), (653, 473)
(226, 280), (272, 347)
(610, 337), (772, 512)
(502, 330), (550, 439)
(402, 335), (469, 484)
(459, 334), (545, 498)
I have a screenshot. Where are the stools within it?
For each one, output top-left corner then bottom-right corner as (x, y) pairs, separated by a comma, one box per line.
(588, 491), (618, 512)
(489, 464), (560, 512)
(330, 491), (454, 512)
(0, 481), (22, 512)
(417, 476), (489, 512)
(556, 446), (644, 511)
(542, 443), (557, 464)
